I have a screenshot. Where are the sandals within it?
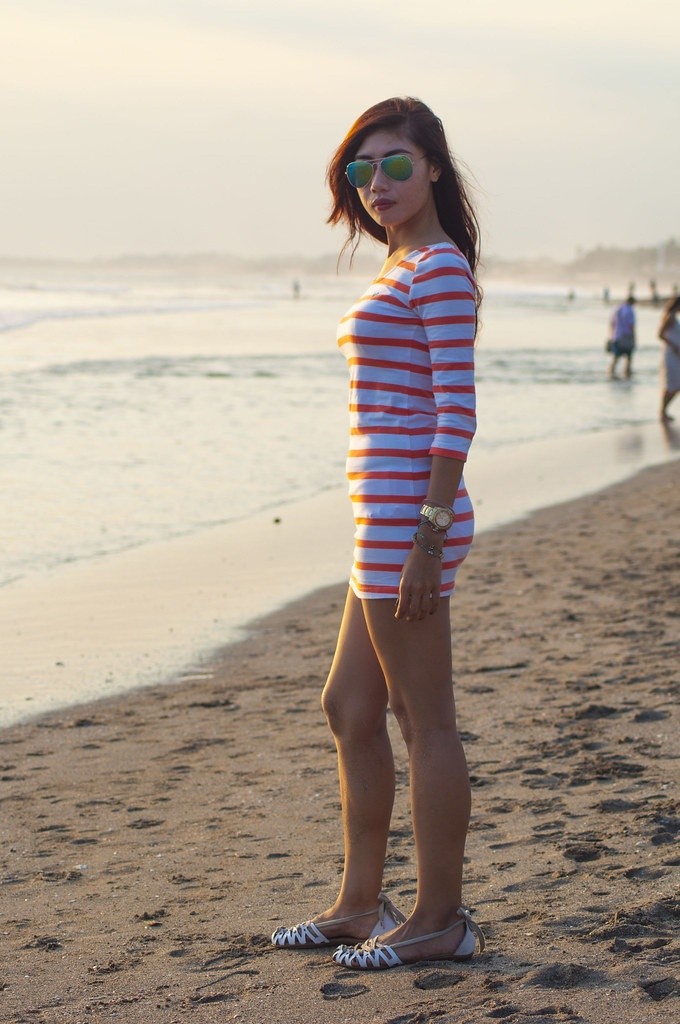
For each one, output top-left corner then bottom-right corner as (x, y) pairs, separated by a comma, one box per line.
(329, 909), (484, 967)
(271, 897), (408, 951)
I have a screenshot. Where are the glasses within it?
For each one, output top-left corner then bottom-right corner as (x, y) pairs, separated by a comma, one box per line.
(341, 150), (431, 189)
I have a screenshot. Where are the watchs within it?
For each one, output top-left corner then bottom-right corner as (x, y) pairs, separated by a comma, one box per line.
(419, 501), (456, 533)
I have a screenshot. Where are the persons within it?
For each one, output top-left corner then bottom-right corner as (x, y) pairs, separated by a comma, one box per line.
(270, 97), (486, 972)
(658, 296), (680, 425)
(605, 296), (636, 381)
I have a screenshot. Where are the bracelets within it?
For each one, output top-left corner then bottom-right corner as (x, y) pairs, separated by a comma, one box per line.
(412, 519), (447, 560)
